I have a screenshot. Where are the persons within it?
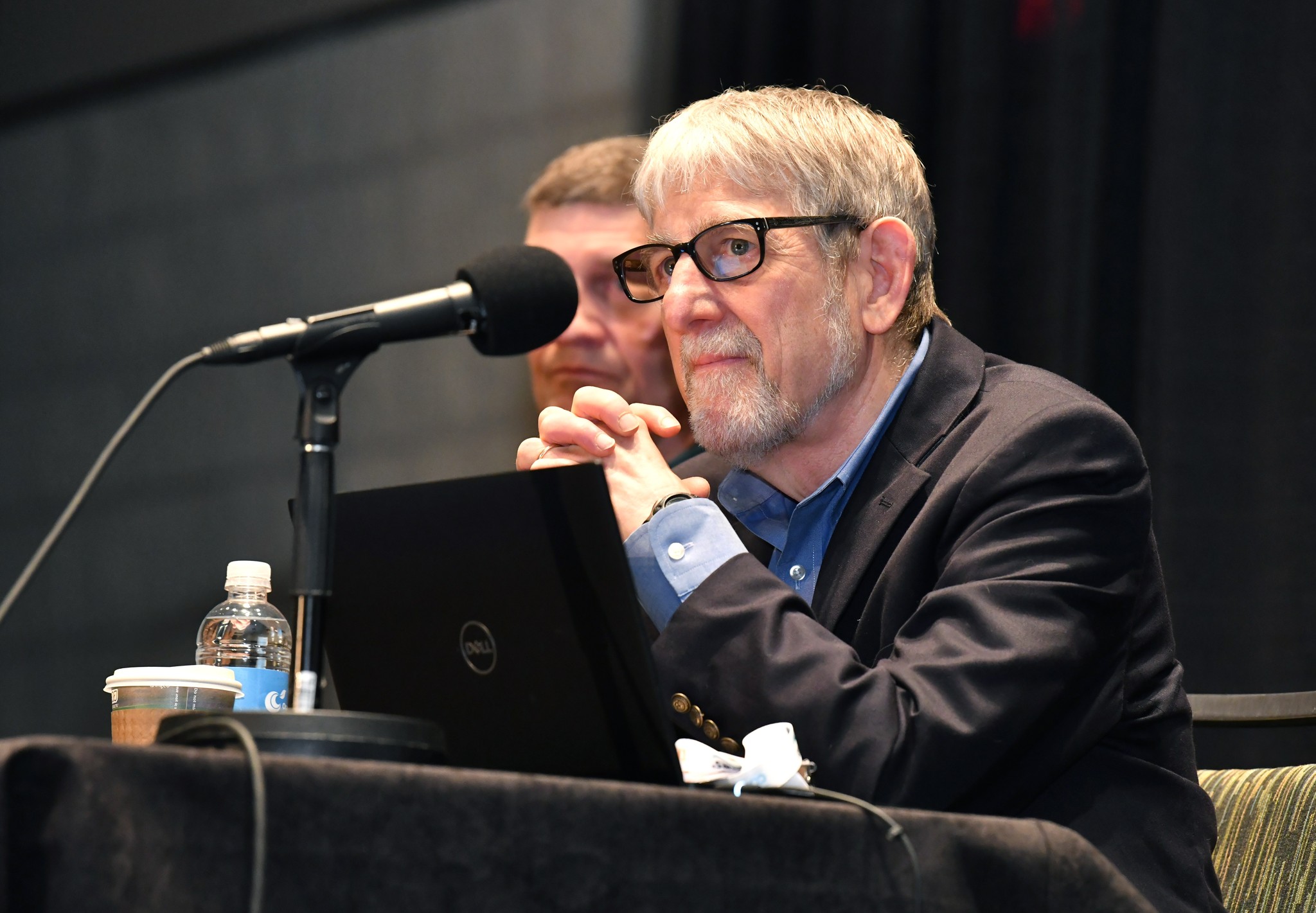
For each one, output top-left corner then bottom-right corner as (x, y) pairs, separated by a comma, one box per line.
(520, 133), (706, 469)
(516, 84), (1229, 913)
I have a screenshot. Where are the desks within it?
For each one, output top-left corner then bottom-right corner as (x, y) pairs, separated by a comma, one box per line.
(0, 732), (1159, 913)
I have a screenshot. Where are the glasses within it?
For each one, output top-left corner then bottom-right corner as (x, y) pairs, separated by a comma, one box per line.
(611, 216), (869, 302)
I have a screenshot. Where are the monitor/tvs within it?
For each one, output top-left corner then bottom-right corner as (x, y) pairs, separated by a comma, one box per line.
(286, 461), (689, 785)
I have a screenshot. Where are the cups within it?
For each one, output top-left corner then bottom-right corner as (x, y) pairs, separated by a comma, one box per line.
(103, 665), (245, 745)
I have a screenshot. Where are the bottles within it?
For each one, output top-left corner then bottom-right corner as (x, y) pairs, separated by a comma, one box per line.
(195, 560), (292, 712)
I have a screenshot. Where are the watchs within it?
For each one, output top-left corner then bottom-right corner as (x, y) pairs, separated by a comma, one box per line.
(643, 492), (698, 524)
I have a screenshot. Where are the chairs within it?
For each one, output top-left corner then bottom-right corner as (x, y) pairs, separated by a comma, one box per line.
(1188, 693), (1316, 913)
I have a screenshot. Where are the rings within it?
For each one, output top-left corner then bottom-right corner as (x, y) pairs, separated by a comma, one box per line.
(538, 444), (562, 460)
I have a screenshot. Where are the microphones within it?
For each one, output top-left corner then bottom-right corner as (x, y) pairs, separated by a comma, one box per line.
(196, 245), (579, 364)
